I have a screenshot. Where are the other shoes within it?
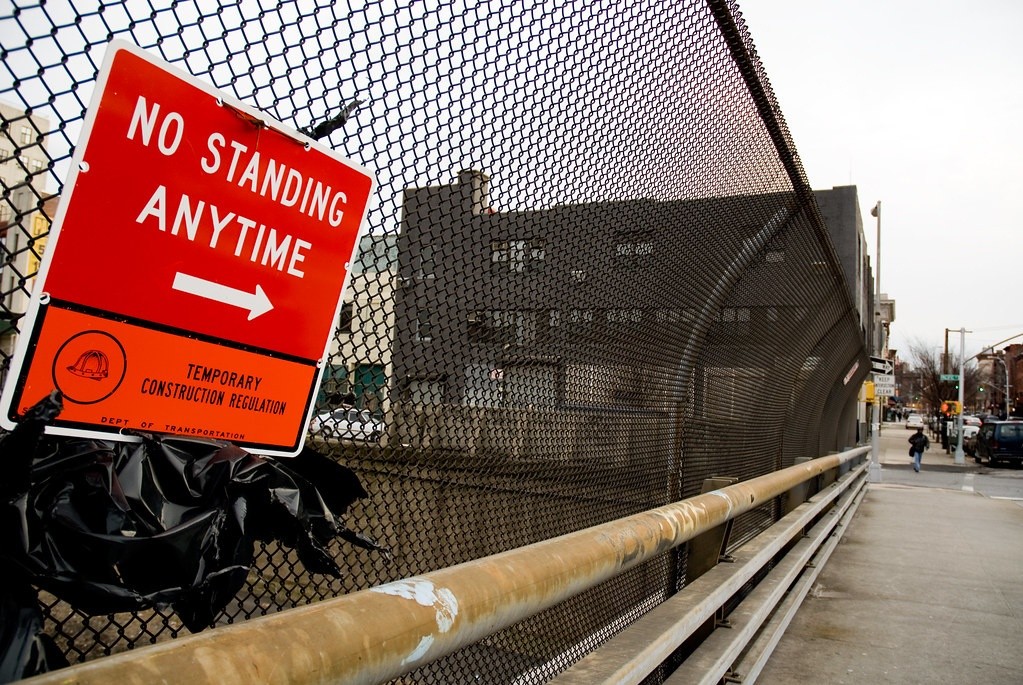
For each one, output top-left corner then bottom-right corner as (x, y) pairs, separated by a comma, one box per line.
(914, 467), (919, 472)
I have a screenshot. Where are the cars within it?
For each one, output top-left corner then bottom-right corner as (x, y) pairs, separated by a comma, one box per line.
(309, 407), (385, 442)
(903, 408), (999, 440)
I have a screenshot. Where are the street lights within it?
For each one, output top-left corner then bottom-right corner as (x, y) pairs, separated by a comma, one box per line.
(987, 356), (1009, 420)
(870, 200), (882, 484)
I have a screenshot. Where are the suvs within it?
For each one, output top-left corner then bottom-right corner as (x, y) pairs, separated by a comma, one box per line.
(975, 421), (1023, 469)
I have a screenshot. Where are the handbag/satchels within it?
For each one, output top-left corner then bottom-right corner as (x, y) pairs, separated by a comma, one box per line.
(909, 447), (915, 457)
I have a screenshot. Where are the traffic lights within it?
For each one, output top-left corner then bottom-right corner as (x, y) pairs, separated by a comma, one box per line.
(956, 381), (959, 389)
(980, 387), (984, 391)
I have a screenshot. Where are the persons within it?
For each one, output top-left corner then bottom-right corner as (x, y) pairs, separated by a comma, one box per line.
(891, 409), (909, 422)
(908, 426), (929, 472)
